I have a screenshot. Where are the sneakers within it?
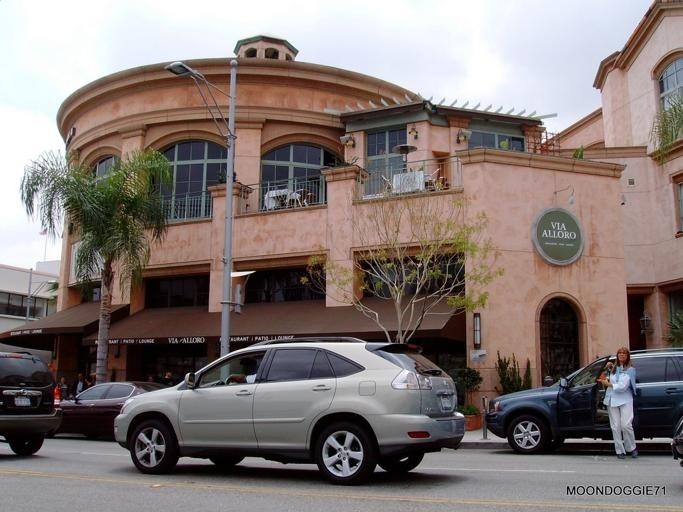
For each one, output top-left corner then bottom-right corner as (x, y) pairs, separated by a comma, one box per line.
(617, 450), (638, 459)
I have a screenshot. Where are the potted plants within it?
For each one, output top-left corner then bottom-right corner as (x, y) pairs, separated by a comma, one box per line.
(457, 404), (481, 431)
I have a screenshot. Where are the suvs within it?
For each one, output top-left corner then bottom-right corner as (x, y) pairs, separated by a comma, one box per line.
(114, 337), (464, 486)
(0, 350), (63, 455)
(485, 348), (682, 459)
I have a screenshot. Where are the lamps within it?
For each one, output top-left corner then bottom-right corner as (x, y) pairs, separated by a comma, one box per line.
(408, 127), (466, 143)
(553, 184), (575, 205)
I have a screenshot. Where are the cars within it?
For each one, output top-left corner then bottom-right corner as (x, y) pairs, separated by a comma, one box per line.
(46, 381), (171, 441)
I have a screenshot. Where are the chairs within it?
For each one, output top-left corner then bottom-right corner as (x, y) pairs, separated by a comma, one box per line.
(263, 189), (317, 212)
(380, 168), (440, 192)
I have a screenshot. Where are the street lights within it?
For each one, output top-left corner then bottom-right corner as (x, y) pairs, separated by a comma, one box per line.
(166, 59), (239, 383)
(26, 269), (56, 318)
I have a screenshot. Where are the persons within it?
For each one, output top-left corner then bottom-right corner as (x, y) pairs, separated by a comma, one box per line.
(599, 347), (640, 460)
(71, 371), (88, 396)
(226, 357), (259, 385)
(84, 375), (94, 388)
(56, 374), (71, 402)
